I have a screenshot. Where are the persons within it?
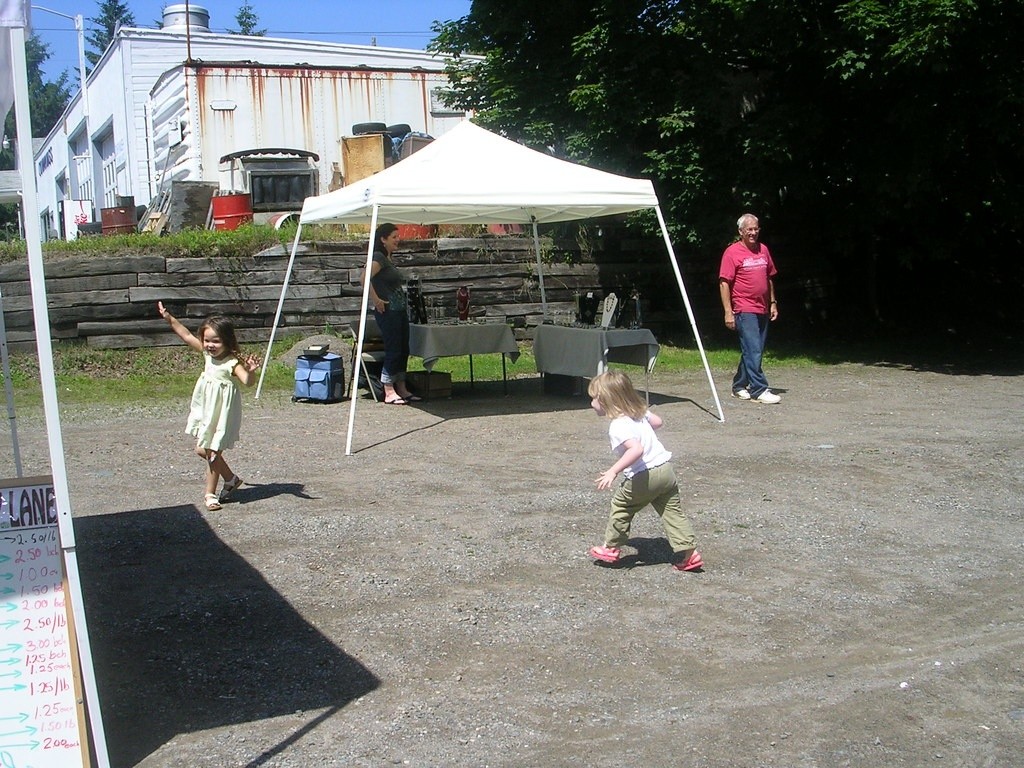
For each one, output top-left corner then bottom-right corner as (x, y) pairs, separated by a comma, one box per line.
(360, 223), (423, 405)
(587, 370), (705, 571)
(719, 214), (784, 404)
(158, 301), (262, 510)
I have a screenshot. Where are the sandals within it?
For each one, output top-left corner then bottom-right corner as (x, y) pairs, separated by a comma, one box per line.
(590, 546), (622, 563)
(204, 494), (221, 511)
(674, 553), (703, 571)
(218, 475), (243, 502)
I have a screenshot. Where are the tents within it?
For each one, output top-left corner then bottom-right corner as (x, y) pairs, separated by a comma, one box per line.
(253, 118), (726, 455)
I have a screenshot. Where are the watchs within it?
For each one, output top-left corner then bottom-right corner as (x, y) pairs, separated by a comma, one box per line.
(770, 300), (777, 304)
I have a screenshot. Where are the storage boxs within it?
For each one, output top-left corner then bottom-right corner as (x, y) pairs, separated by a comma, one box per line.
(406, 370), (451, 401)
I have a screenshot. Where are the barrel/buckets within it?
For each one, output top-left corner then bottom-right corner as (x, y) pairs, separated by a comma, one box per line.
(172, 181), (220, 235)
(211, 193), (254, 232)
(100, 206), (138, 237)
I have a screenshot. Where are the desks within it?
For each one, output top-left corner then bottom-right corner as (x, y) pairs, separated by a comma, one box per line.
(408, 322), (521, 398)
(533, 323), (660, 407)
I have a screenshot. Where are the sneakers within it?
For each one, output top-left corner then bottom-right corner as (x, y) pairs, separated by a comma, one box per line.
(731, 389), (751, 400)
(750, 389), (781, 404)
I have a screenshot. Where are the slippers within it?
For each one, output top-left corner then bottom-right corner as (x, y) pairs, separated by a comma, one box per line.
(405, 395), (423, 402)
(385, 397), (410, 405)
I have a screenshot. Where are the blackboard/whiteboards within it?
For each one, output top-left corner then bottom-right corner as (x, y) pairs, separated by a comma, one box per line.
(0, 473), (100, 768)
